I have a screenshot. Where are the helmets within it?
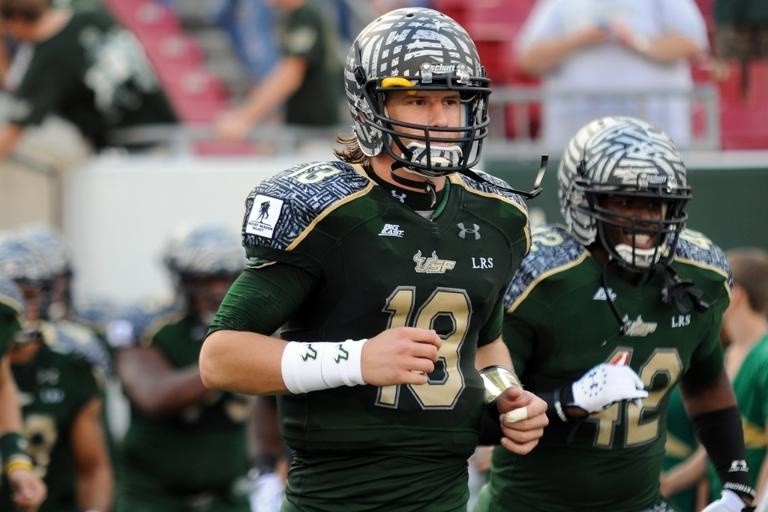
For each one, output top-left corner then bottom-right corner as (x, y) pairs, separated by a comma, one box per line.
(342, 7), (491, 157)
(4, 221), (73, 288)
(165, 222), (246, 281)
(558, 115), (695, 246)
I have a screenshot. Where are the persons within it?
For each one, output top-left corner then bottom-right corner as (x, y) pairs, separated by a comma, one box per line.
(1, 225), (285, 512)
(509, 0), (708, 147)
(198, 7), (767, 512)
(0, 0), (342, 161)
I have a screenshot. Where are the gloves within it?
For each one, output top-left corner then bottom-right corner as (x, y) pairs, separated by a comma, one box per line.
(562, 363), (649, 422)
